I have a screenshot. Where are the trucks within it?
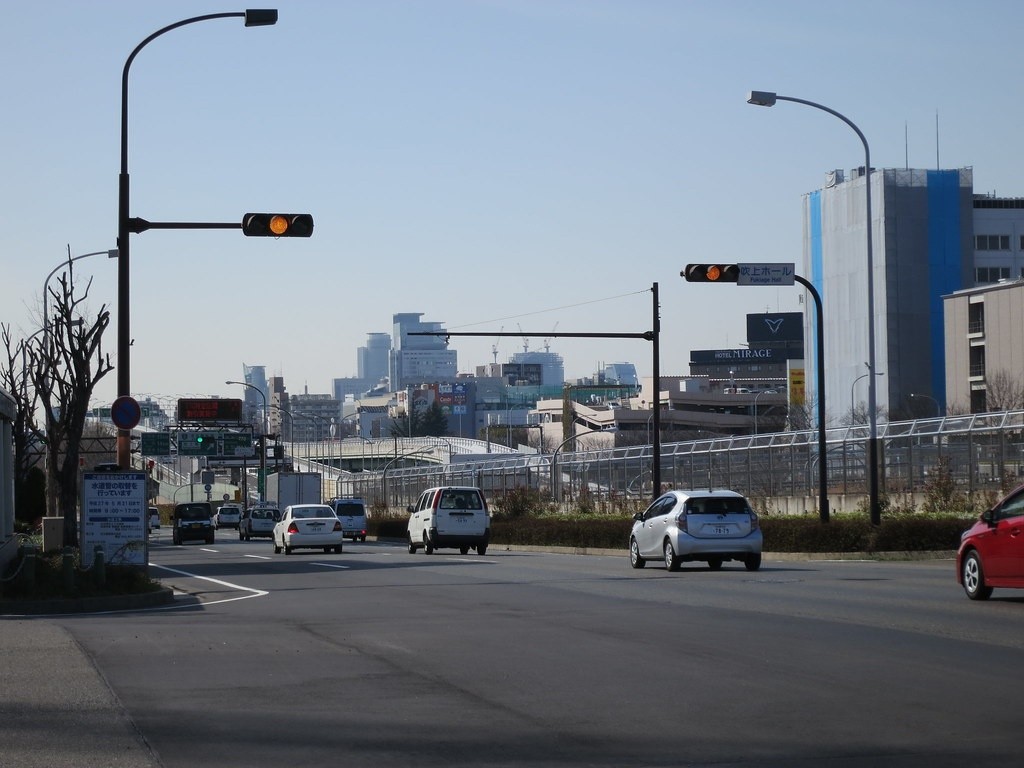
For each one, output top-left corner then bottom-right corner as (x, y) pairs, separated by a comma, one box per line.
(266, 473), (322, 517)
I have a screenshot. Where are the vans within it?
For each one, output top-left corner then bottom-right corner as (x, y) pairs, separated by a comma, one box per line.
(239, 507), (280, 542)
(406, 486), (490, 555)
(169, 502), (215, 545)
(212, 507), (241, 530)
(148, 507), (161, 533)
(329, 499), (366, 543)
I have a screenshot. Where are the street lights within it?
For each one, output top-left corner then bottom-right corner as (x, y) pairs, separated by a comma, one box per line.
(117, 7), (278, 477)
(510, 404), (536, 453)
(224, 381), (267, 503)
(755, 386), (786, 468)
(746, 89), (881, 524)
(9, 320), (80, 395)
(573, 414), (598, 452)
(551, 425), (619, 501)
(41, 250), (116, 517)
(850, 371), (884, 476)
(252, 403), (451, 505)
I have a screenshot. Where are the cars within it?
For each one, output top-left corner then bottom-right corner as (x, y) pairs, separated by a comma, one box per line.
(955, 485), (1023, 599)
(159, 455), (177, 464)
(273, 504), (344, 554)
(630, 487), (763, 572)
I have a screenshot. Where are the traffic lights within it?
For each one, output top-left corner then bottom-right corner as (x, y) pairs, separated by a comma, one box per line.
(686, 264), (741, 284)
(197, 437), (214, 442)
(243, 213), (312, 238)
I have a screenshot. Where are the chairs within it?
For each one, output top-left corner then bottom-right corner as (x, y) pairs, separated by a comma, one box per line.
(453, 497), (468, 508)
